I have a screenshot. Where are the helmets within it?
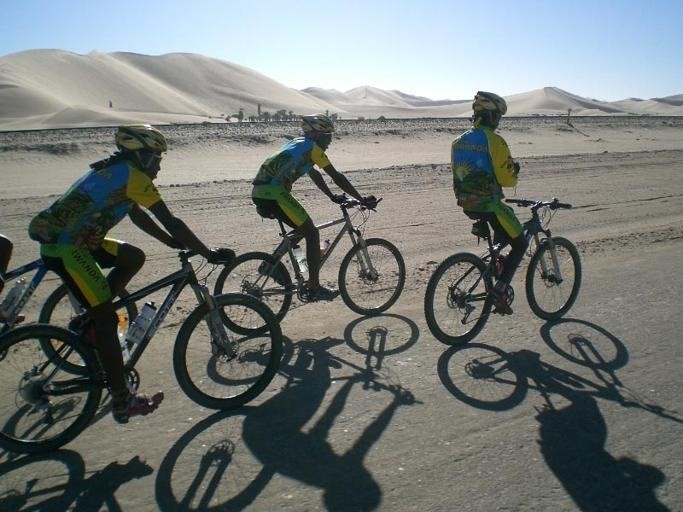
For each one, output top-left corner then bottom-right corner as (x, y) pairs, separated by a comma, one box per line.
(115, 125), (167, 152)
(471, 92), (507, 115)
(301, 114), (335, 135)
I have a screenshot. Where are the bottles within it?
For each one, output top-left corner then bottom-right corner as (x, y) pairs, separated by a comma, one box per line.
(125, 301), (157, 345)
(119, 333), (131, 366)
(293, 244), (308, 273)
(319, 239), (331, 258)
(1, 277), (26, 318)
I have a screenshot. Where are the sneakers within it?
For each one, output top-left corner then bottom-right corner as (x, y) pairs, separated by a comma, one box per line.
(112, 391), (164, 424)
(259, 261), (287, 284)
(306, 286), (339, 300)
(490, 284), (514, 315)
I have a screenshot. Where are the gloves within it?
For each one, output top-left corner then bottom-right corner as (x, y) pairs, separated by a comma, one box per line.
(332, 193), (346, 204)
(208, 248), (235, 267)
(361, 195), (377, 209)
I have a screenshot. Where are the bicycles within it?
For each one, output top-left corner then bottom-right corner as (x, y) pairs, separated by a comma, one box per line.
(0, 244), (284, 456)
(203, 310), (431, 407)
(0, 230), (139, 383)
(0, 402), (294, 512)
(210, 193), (407, 336)
(423, 194), (584, 346)
(434, 316), (682, 429)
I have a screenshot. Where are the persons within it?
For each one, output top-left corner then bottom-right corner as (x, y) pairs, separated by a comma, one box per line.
(451, 90), (529, 314)
(0, 234), (25, 325)
(251, 114), (377, 300)
(29, 124), (235, 422)
